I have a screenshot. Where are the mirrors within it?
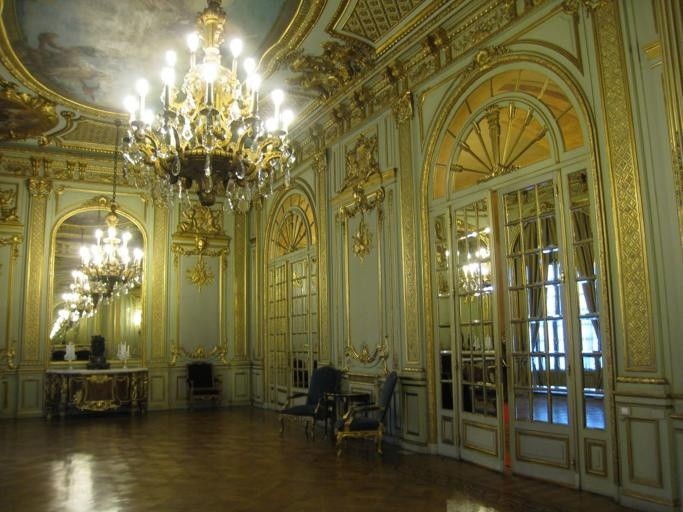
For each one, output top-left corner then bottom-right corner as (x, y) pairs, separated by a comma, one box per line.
(45, 195), (149, 366)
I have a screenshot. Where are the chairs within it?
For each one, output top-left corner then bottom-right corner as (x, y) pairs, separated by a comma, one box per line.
(184, 361), (225, 412)
(278, 365), (398, 457)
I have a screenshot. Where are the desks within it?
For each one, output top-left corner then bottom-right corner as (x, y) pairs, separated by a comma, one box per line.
(45, 368), (148, 419)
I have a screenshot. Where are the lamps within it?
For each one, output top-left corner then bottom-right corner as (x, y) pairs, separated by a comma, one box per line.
(48, 214), (143, 340)
(119, 0), (297, 215)
